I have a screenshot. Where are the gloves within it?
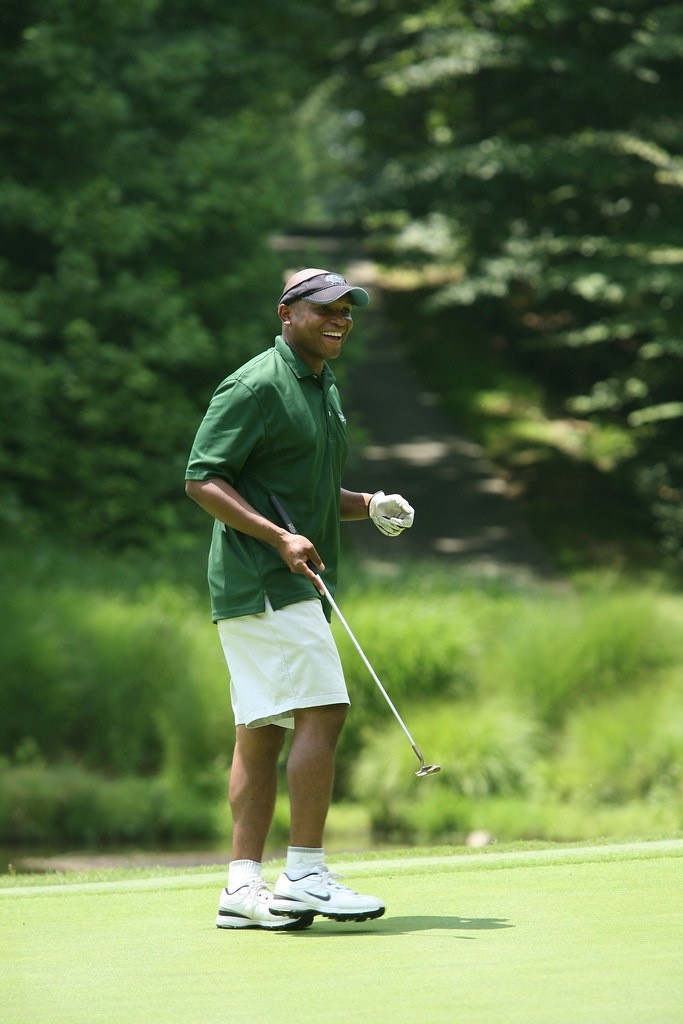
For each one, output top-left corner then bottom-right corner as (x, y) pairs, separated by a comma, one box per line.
(368, 490), (415, 537)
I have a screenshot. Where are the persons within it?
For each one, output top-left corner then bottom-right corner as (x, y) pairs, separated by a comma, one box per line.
(184, 267), (416, 934)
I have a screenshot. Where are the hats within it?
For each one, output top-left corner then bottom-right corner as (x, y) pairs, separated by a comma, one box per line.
(280, 267), (370, 308)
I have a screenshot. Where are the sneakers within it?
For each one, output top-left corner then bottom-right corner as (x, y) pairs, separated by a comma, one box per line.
(216, 866), (385, 930)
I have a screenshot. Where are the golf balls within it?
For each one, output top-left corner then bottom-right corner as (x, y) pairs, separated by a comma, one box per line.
(466, 830), (490, 848)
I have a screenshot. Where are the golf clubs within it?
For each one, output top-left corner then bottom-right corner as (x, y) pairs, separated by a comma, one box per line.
(264, 484), (441, 778)
